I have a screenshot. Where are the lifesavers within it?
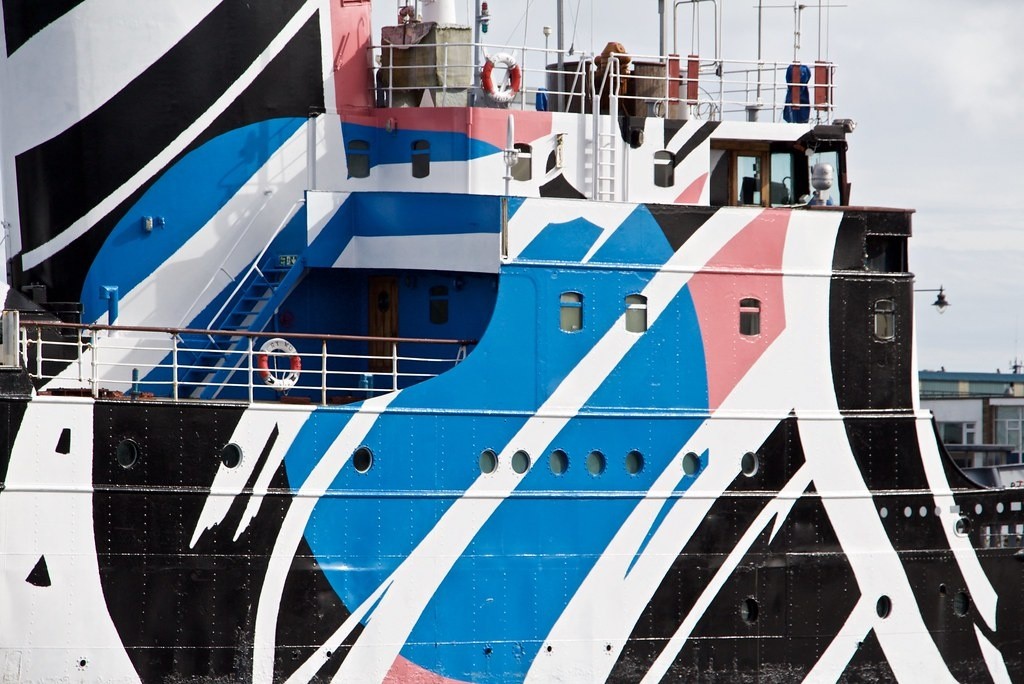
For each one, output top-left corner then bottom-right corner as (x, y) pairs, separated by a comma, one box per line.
(482, 54), (521, 102)
(257, 338), (304, 387)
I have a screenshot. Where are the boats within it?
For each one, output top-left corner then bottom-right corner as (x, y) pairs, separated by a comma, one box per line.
(0, 1), (1024, 684)
